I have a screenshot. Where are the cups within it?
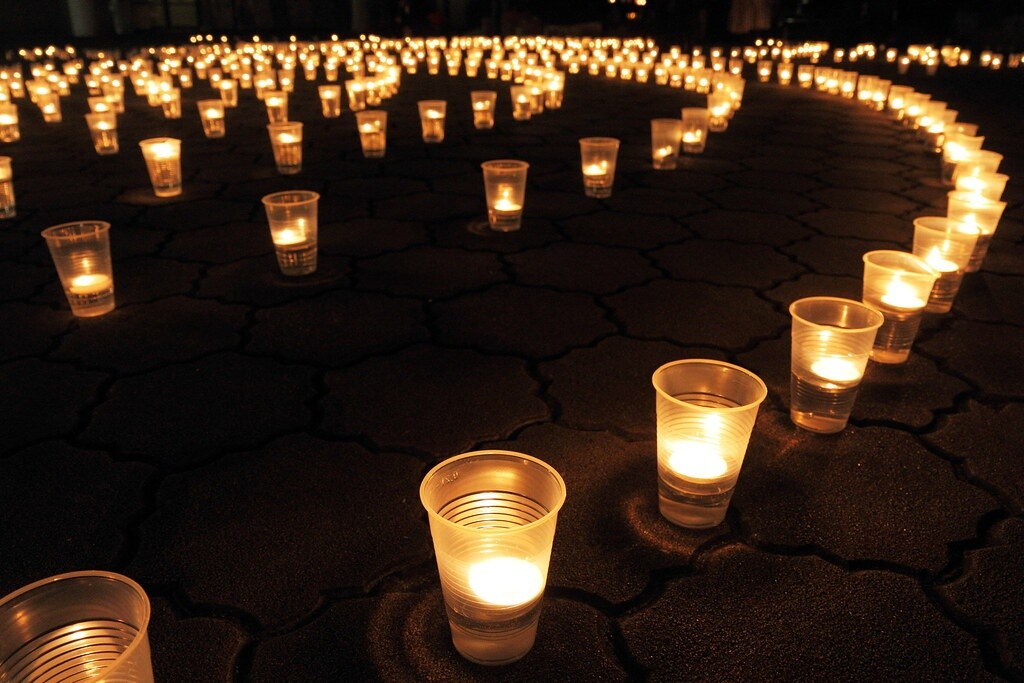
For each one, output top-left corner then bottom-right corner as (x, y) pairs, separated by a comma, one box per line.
(860, 251), (942, 364)
(956, 171), (1009, 200)
(421, 451), (566, 667)
(40, 221), (117, 318)
(787, 298), (885, 435)
(912, 217), (981, 313)
(622, 36), (1024, 187)
(946, 189), (1007, 272)
(0, 571), (152, 683)
(263, 187), (321, 273)
(0, 34), (621, 230)
(652, 360), (768, 528)
